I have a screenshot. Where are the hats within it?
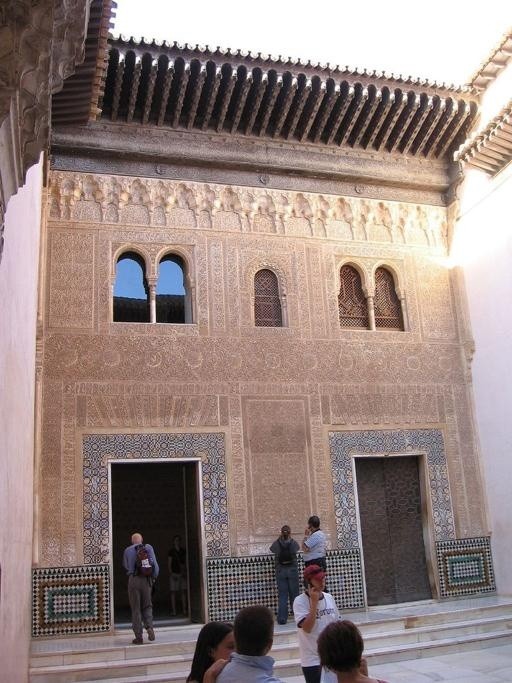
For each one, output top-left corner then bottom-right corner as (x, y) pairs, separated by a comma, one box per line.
(304, 566), (326, 580)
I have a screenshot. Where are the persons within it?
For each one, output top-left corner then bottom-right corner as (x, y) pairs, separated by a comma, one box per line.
(301, 516), (327, 591)
(216, 604), (286, 683)
(167, 536), (189, 617)
(292, 566), (340, 683)
(186, 621), (235, 683)
(270, 525), (300, 623)
(123, 532), (160, 644)
(315, 619), (389, 683)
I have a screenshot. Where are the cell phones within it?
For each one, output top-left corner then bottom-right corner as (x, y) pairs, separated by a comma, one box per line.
(309, 525), (312, 529)
(303, 579), (312, 588)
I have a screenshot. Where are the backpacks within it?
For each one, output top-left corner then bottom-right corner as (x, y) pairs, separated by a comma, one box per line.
(132, 544), (154, 577)
(276, 539), (293, 564)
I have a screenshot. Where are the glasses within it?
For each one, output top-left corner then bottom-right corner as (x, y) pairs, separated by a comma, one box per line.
(307, 568), (323, 574)
